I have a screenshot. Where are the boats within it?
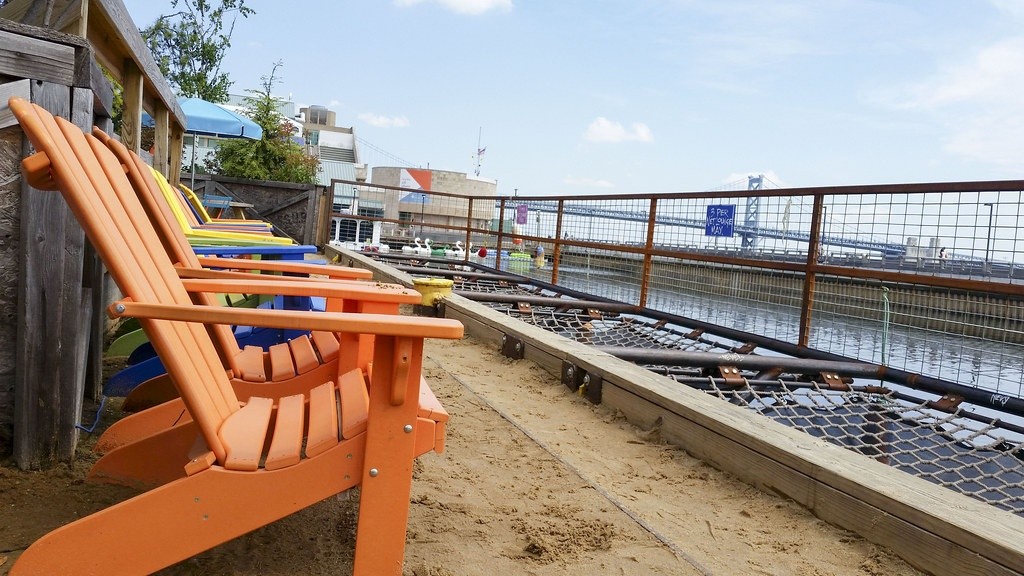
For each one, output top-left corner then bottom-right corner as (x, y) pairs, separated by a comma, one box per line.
(403, 238), (532, 262)
(330, 240), (390, 254)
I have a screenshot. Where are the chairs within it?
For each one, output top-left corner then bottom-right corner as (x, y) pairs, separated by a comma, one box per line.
(8, 97), (464, 576)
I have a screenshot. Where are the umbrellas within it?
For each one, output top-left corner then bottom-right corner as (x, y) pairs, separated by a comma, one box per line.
(142, 98), (263, 191)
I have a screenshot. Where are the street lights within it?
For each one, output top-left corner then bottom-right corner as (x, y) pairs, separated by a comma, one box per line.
(982, 202), (994, 282)
